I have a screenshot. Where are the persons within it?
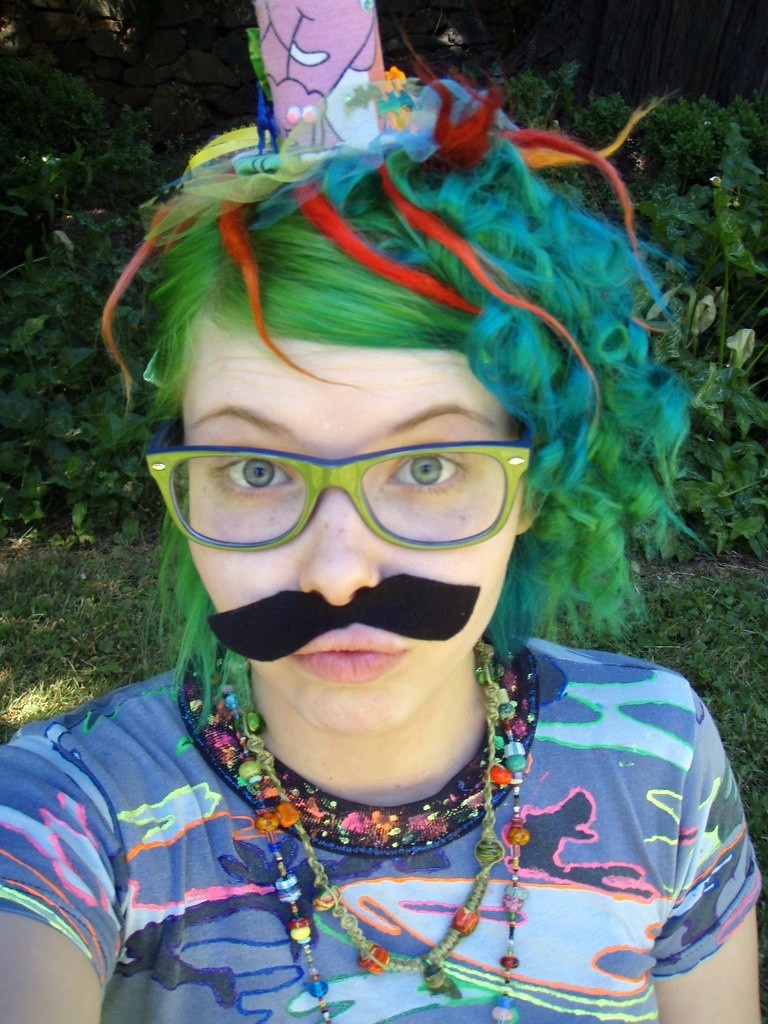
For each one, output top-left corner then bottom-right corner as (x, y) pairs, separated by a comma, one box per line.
(0, 78), (763, 1024)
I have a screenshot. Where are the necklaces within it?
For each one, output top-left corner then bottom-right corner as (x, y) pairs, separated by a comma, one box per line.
(215, 639), (527, 1024)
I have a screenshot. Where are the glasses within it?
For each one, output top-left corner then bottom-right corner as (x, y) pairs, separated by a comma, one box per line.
(143, 421), (532, 551)
